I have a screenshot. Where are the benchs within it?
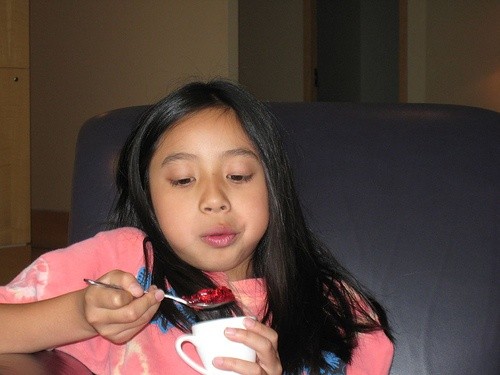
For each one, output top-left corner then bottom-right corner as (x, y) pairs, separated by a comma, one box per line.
(67, 101), (500, 375)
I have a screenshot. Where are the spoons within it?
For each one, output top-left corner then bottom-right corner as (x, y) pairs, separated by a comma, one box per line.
(83, 278), (238, 312)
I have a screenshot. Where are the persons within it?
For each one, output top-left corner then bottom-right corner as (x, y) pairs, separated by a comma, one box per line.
(0, 77), (396, 375)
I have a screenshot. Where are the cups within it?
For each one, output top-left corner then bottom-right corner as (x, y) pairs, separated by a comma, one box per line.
(175, 316), (257, 375)
